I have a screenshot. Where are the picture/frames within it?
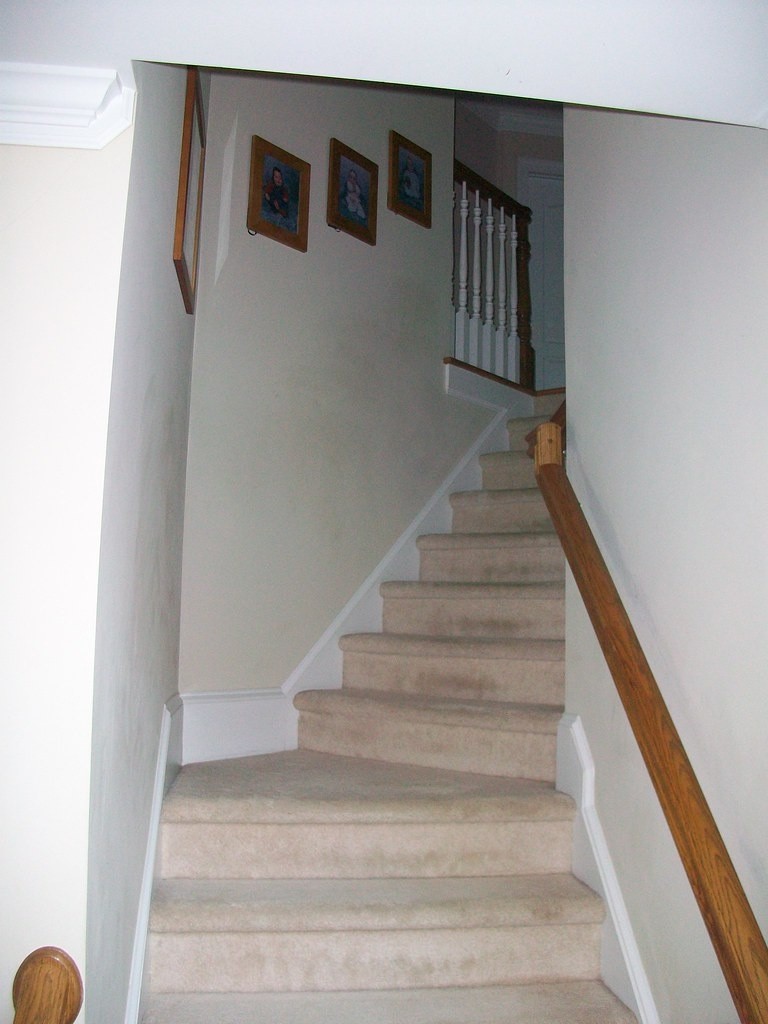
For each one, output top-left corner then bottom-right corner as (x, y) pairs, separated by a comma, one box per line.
(386, 130), (432, 229)
(173, 66), (207, 315)
(247, 134), (312, 253)
(326, 138), (379, 247)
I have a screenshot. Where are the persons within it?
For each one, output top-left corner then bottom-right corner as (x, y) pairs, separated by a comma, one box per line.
(345, 165), (367, 220)
(264, 167), (290, 218)
(402, 156), (420, 199)
